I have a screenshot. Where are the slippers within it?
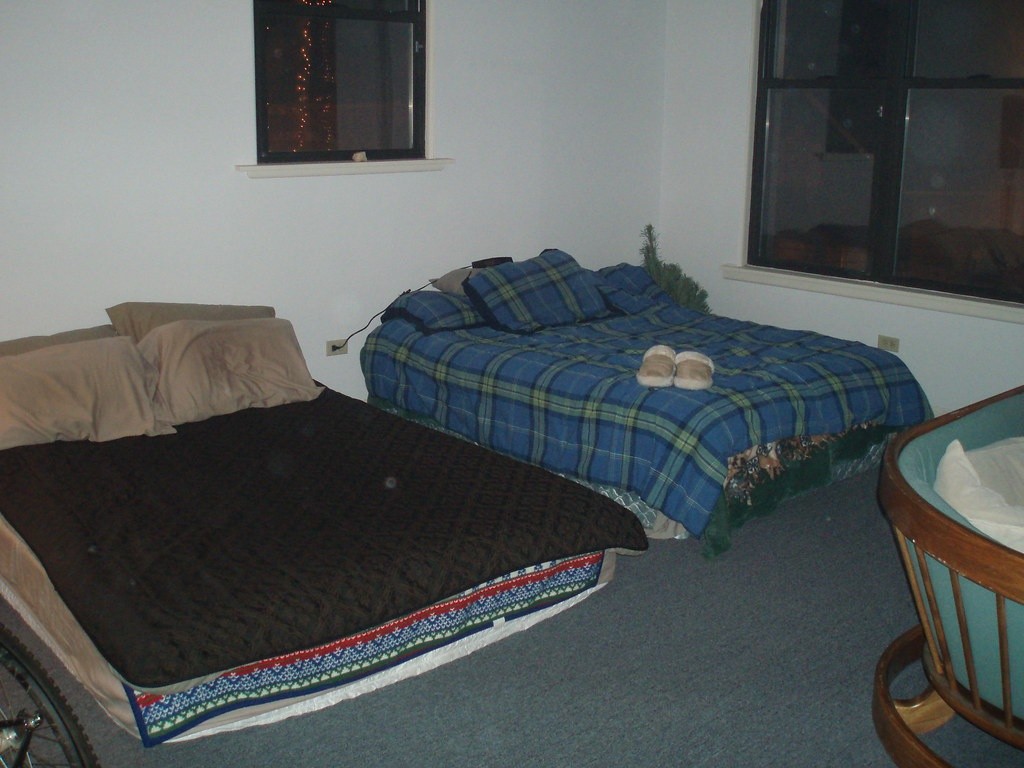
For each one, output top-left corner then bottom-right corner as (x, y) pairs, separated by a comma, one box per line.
(636, 346), (715, 390)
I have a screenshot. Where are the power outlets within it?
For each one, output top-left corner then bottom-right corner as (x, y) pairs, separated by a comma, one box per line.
(326, 338), (348, 357)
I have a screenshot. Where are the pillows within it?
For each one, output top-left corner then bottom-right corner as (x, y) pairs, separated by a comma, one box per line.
(137, 317), (327, 430)
(380, 291), (488, 334)
(467, 248), (611, 335)
(105, 302), (275, 340)
(0, 334), (178, 451)
(428, 267), (474, 301)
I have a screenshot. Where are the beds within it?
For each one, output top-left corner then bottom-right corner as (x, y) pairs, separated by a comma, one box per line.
(870, 384), (1024, 768)
(0, 325), (649, 750)
(358, 260), (939, 565)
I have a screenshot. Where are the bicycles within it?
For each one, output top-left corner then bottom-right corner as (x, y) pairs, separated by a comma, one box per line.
(0, 625), (100, 768)
